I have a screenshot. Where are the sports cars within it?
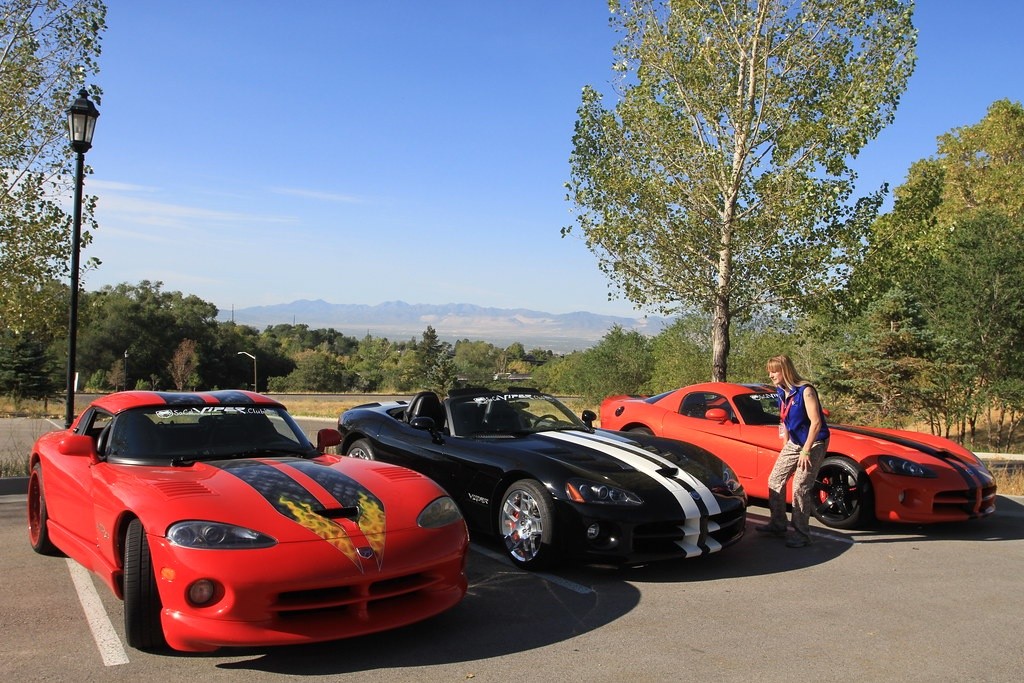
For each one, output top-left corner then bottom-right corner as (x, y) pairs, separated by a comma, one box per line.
(336, 387), (748, 574)
(600, 380), (997, 531)
(27, 388), (472, 656)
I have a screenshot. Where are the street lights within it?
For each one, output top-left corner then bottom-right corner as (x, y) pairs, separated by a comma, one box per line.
(237, 351), (257, 392)
(63, 85), (102, 432)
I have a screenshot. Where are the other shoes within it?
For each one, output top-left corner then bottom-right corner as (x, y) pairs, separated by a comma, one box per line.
(787, 531), (809, 546)
(755, 523), (788, 534)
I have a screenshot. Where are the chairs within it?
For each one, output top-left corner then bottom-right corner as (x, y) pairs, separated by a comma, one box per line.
(453, 399), (524, 436)
(731, 394), (763, 420)
(162, 423), (265, 450)
(407, 391), (446, 427)
(681, 393), (707, 418)
(97, 414), (159, 456)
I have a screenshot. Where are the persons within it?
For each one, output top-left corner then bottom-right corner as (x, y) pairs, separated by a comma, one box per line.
(755, 354), (830, 547)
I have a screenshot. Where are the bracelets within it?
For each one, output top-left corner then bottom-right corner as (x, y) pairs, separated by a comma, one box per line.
(801, 449), (810, 456)
